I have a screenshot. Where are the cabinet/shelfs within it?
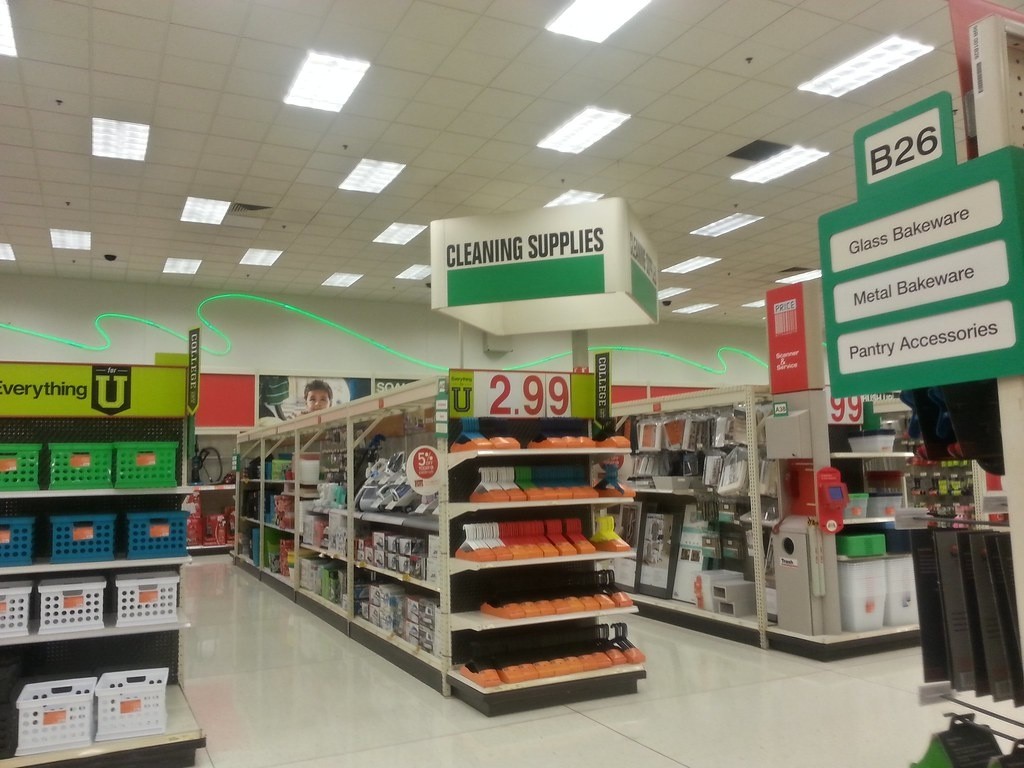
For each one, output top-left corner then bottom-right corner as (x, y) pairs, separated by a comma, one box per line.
(228, 352), (648, 718)
(594, 279), (919, 650)
(874, 399), (1011, 532)
(187, 427), (255, 549)
(0, 324), (207, 768)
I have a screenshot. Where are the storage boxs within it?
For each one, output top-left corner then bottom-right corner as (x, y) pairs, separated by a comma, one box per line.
(428, 534), (440, 559)
(38, 577), (107, 635)
(110, 570), (180, 627)
(48, 443), (114, 490)
(94, 667), (170, 741)
(0, 516), (37, 567)
(361, 601), (369, 620)
(369, 603), (380, 626)
(126, 511), (191, 559)
(356, 532), (425, 580)
(14, 677), (99, 757)
(0, 443), (43, 492)
(426, 560), (439, 585)
(113, 441), (179, 489)
(369, 583), (379, 606)
(300, 558), (347, 607)
(379, 584), (440, 655)
(302, 513), (348, 558)
(49, 515), (114, 565)
(0, 579), (35, 638)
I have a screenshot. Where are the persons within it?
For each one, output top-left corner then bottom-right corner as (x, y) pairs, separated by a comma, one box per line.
(297, 380), (333, 415)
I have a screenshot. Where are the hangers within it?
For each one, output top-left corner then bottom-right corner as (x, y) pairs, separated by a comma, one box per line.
(455, 519), (596, 562)
(450, 416), (521, 453)
(459, 622), (645, 687)
(593, 464), (636, 498)
(469, 465), (598, 502)
(527, 417), (632, 448)
(480, 569), (634, 620)
(589, 516), (631, 552)
